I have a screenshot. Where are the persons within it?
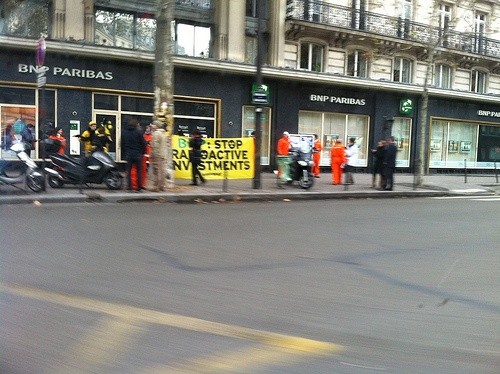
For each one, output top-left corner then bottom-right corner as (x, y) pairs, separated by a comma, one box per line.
(49, 127), (66, 170)
(277, 131), (292, 176)
(78, 119), (168, 193)
(20, 124), (37, 157)
(331, 136), (398, 191)
(0, 125), (15, 151)
(310, 134), (323, 178)
(188, 129), (207, 186)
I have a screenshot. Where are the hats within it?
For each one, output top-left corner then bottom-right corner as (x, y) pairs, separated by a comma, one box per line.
(153, 120), (164, 128)
(89, 120), (96, 126)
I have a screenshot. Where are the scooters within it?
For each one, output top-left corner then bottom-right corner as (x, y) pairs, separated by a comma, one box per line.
(0, 140), (45, 193)
(42, 133), (124, 191)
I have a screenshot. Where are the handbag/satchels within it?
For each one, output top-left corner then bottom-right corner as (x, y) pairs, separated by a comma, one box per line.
(22, 140), (35, 150)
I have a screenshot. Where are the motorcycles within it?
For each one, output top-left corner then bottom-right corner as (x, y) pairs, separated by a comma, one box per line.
(288, 139), (319, 190)
